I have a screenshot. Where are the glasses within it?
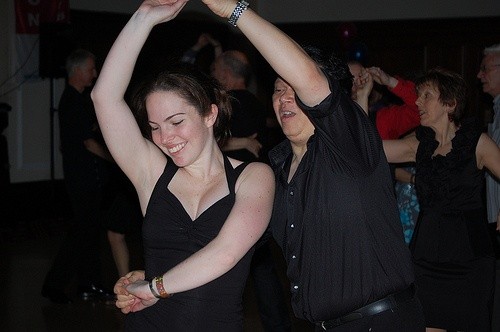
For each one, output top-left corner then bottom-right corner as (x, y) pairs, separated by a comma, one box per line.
(481, 64), (500, 72)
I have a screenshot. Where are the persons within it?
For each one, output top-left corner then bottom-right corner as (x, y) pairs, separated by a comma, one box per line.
(356, 68), (500, 332)
(90, 1), (277, 332)
(57, 30), (500, 282)
(114, 0), (427, 332)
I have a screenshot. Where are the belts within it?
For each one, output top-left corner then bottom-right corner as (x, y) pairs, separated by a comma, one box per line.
(309, 283), (415, 332)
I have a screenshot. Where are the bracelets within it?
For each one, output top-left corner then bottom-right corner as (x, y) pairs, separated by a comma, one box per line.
(214, 44), (222, 48)
(227, 0), (250, 27)
(411, 173), (415, 185)
(149, 274), (173, 299)
